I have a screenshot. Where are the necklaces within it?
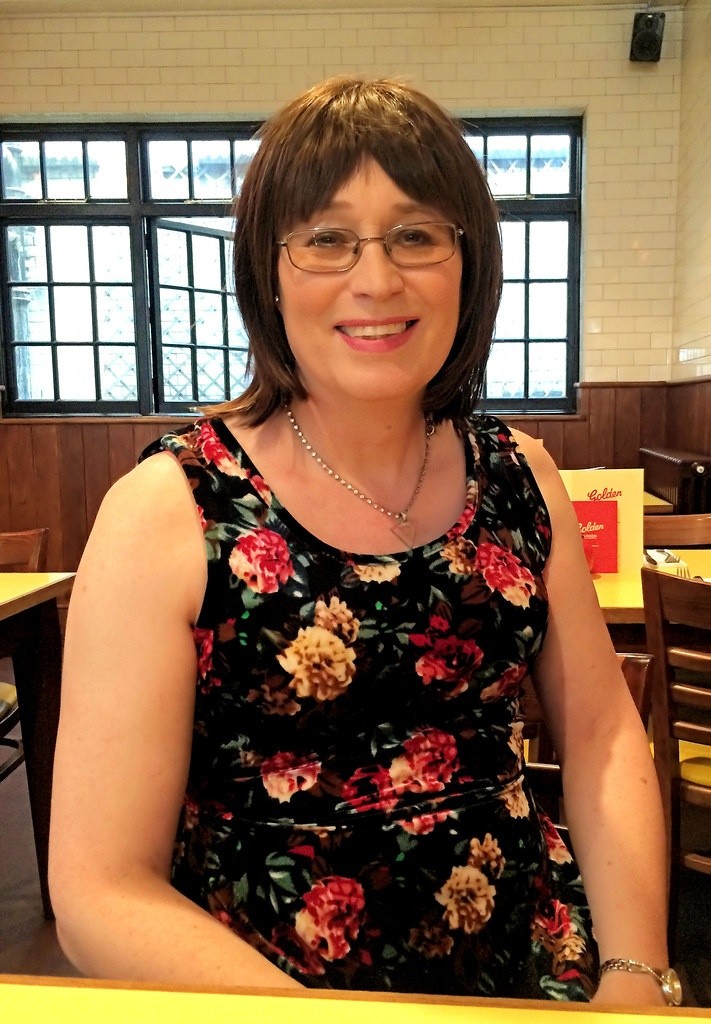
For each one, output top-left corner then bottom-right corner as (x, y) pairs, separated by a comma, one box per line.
(278, 388), (445, 553)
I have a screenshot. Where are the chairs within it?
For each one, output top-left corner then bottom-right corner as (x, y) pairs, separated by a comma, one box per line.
(0, 528), (49, 782)
(517, 514), (711, 968)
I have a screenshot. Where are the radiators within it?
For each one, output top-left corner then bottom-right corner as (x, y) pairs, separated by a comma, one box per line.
(639, 448), (711, 515)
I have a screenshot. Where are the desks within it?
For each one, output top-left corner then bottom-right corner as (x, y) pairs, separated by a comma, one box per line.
(0, 573), (79, 920)
(0, 974), (711, 1024)
(644, 491), (673, 513)
(590, 550), (711, 624)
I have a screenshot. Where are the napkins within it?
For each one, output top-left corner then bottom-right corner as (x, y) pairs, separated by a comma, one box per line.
(645, 549), (686, 566)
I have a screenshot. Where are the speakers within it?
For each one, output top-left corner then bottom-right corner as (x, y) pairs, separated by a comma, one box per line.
(628, 10), (665, 63)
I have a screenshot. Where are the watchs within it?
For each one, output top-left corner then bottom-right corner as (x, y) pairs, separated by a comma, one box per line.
(590, 955), (684, 1007)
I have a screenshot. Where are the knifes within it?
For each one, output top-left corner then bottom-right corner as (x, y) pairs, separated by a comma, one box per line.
(643, 548), (657, 565)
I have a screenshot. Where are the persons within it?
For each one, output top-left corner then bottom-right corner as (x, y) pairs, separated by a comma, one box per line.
(49, 77), (685, 1013)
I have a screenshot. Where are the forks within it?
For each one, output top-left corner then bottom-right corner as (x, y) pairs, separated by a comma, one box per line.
(677, 567), (690, 579)
(656, 548), (680, 563)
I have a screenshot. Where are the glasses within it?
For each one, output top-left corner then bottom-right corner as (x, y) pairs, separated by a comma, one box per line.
(272, 220), (463, 274)
